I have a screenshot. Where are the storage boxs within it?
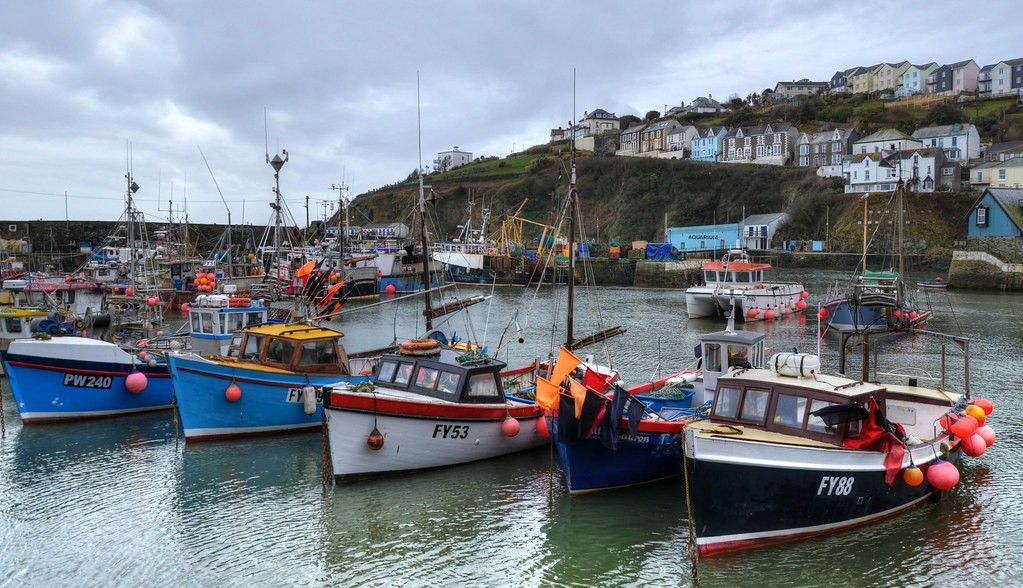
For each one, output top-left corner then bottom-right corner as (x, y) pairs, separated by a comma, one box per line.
(635, 390), (695, 412)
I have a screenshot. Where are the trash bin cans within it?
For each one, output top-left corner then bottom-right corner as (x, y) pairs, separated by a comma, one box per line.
(684, 147), (691, 158)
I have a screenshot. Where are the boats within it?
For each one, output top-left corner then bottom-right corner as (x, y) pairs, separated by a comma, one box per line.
(3, 105), (341, 425)
(685, 204), (808, 323)
(917, 276), (948, 288)
(681, 329), (995, 560)
(163, 252), (487, 447)
(0, 139), (515, 377)
(535, 289), (767, 495)
(815, 141), (935, 335)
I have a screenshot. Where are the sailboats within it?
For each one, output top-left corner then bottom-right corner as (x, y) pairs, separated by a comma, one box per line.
(319, 68), (625, 486)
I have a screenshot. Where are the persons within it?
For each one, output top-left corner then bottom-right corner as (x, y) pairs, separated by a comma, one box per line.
(790, 242), (795, 253)
(731, 346), (747, 366)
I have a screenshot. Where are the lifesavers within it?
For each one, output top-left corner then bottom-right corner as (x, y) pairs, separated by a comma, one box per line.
(229, 298), (251, 307)
(400, 338), (441, 356)
